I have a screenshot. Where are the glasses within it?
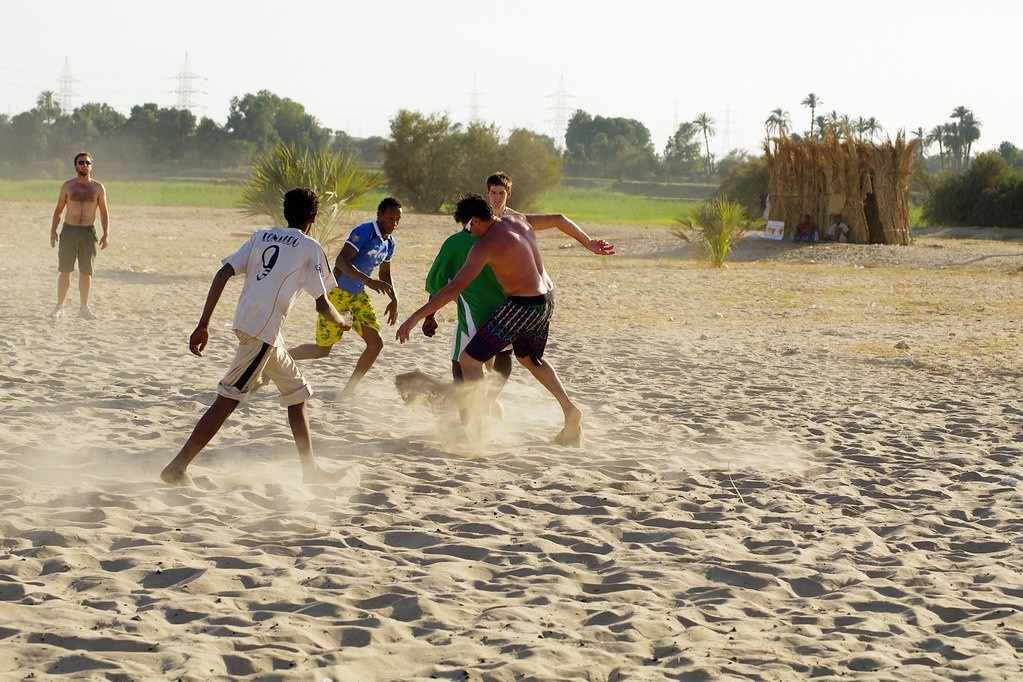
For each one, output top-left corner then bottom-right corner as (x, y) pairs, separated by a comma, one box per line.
(463, 218), (473, 235)
(76, 160), (92, 166)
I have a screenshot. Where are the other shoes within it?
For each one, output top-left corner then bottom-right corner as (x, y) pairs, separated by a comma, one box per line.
(79, 306), (98, 320)
(48, 305), (64, 318)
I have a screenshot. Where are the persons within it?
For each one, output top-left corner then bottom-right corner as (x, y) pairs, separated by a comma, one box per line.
(286, 198), (403, 400)
(395, 171), (616, 442)
(48, 152), (108, 320)
(160, 188), (352, 489)
